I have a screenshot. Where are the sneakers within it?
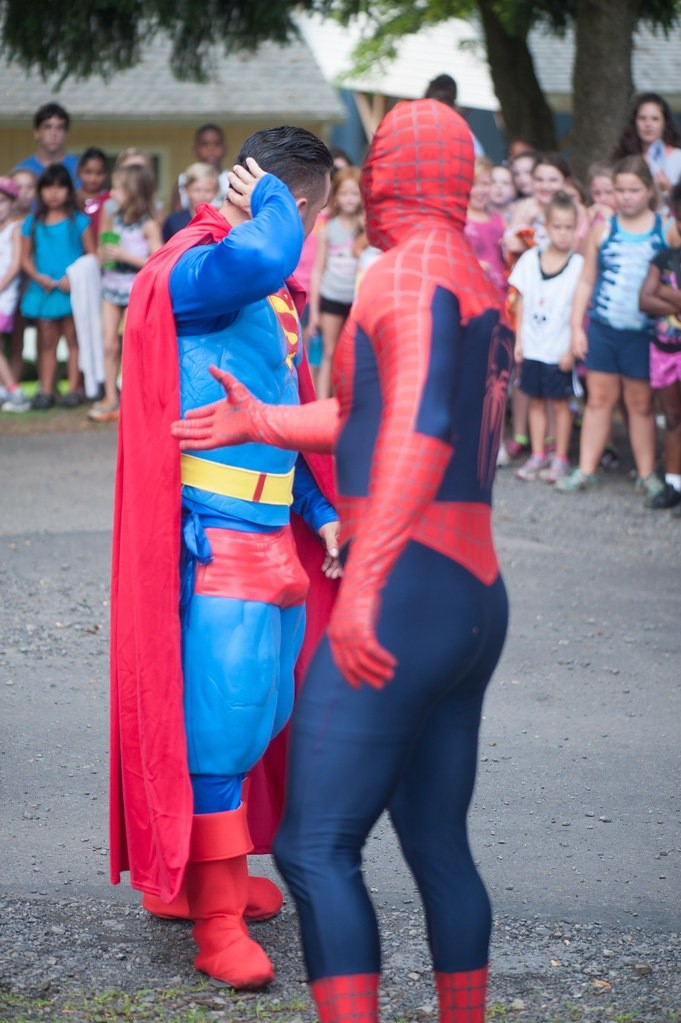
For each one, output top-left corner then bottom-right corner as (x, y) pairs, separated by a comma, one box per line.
(538, 459), (571, 484)
(557, 468), (594, 492)
(635, 473), (666, 496)
(516, 455), (548, 480)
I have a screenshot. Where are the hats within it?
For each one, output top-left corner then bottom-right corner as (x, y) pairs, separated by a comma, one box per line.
(0, 176), (18, 199)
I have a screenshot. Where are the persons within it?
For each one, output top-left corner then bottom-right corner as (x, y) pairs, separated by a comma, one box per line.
(108, 125), (339, 989)
(290, 148), (384, 399)
(424, 74), (681, 507)
(168, 100), (510, 1023)
(0, 101), (232, 420)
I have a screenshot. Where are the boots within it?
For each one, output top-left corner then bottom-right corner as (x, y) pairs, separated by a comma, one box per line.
(142, 858), (283, 918)
(189, 800), (275, 987)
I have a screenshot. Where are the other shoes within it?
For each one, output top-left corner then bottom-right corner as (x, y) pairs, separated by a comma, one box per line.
(2, 393), (32, 412)
(58, 389), (84, 407)
(85, 399), (121, 420)
(33, 392), (62, 409)
(544, 437), (557, 451)
(649, 485), (681, 507)
(504, 437), (529, 456)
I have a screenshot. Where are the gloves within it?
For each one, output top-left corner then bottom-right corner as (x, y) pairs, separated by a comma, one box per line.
(170, 365), (268, 450)
(326, 575), (398, 692)
